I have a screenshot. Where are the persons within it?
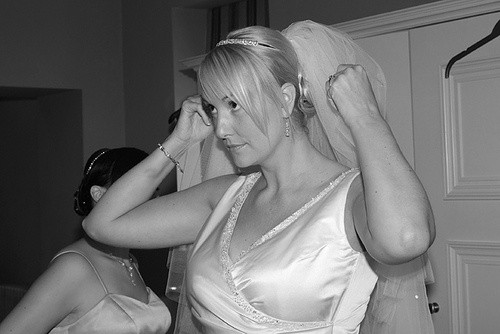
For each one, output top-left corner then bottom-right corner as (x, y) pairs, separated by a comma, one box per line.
(79, 19), (438, 334)
(1, 149), (173, 334)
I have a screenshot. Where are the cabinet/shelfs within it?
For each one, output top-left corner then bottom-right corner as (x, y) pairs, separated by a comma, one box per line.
(179, 1), (500, 334)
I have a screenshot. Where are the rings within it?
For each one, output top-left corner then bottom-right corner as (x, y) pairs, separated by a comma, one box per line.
(329, 76), (336, 88)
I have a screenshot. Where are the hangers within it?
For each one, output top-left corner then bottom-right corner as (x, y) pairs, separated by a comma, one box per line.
(445, 19), (500, 79)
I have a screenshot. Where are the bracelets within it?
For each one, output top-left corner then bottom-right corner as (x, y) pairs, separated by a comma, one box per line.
(158, 144), (185, 175)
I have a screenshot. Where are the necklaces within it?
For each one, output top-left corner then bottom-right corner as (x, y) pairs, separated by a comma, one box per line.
(106, 246), (138, 289)
